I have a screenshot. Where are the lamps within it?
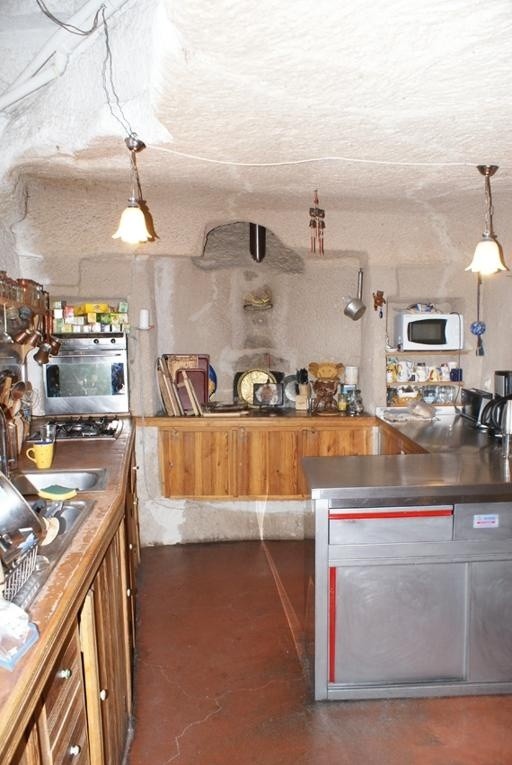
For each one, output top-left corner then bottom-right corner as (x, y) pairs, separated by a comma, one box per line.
(111, 135), (154, 244)
(463, 163), (509, 277)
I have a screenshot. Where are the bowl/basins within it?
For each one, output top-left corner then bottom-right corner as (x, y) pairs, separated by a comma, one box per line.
(424, 396), (435, 403)
(0, 471), (42, 538)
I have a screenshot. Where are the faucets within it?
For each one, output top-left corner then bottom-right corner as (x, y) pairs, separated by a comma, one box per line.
(1, 406), (16, 476)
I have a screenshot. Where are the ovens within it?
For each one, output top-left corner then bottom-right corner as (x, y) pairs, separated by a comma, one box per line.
(18, 333), (128, 415)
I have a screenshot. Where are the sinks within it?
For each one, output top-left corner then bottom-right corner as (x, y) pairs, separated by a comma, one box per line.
(8, 468), (109, 495)
(26, 497), (97, 535)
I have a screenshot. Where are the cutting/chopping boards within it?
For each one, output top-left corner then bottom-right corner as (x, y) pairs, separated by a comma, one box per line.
(167, 354), (197, 383)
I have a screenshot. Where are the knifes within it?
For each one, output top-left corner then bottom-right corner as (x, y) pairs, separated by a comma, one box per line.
(295, 368), (310, 385)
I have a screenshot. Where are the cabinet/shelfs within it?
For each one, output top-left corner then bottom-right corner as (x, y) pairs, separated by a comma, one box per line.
(384, 350), (464, 407)
(9, 520), (133, 764)
(158, 425), (374, 502)
(326, 501), (510, 689)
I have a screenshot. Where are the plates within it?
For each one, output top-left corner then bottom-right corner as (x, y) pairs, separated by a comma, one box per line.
(238, 369), (297, 408)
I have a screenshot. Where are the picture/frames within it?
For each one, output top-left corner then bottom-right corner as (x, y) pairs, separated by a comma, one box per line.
(251, 383), (283, 406)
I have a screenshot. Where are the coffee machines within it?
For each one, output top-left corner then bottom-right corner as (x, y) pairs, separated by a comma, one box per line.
(483, 371), (512, 438)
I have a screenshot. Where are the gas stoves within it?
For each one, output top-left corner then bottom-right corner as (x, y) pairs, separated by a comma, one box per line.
(28, 416), (120, 442)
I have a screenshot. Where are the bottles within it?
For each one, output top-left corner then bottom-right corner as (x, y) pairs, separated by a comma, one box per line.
(0, 406), (17, 480)
(338, 390), (364, 417)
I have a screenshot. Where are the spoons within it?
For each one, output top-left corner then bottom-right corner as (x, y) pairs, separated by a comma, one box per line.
(8, 382), (25, 418)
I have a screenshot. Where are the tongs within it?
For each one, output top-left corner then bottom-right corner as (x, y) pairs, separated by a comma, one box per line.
(157, 358), (203, 417)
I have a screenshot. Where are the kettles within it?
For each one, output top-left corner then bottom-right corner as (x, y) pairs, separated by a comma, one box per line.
(492, 393), (512, 441)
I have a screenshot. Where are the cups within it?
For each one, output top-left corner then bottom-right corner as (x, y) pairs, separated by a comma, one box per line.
(15, 328), (60, 365)
(24, 425), (56, 469)
(501, 434), (512, 459)
(387, 360), (463, 382)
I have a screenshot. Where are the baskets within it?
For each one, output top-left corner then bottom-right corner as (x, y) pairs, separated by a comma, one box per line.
(0, 540), (38, 601)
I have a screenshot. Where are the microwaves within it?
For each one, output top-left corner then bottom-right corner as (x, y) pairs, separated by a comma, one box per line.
(395, 314), (465, 352)
